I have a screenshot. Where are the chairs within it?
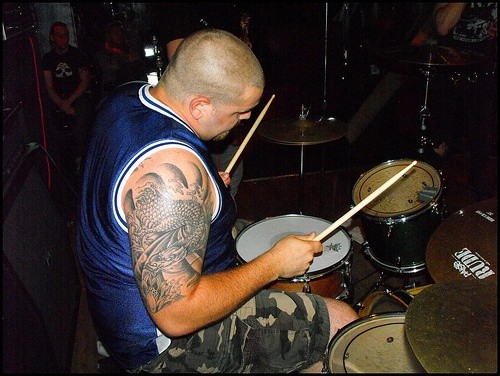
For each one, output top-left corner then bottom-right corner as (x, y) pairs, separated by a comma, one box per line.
(0, 153), (128, 376)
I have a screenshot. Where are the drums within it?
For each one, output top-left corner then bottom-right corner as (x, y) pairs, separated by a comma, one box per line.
(352, 157), (447, 274)
(358, 284), (434, 319)
(321, 311), (426, 373)
(233, 213), (354, 306)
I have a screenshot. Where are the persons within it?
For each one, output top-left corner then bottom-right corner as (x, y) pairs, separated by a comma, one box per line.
(76, 28), (358, 373)
(159, 0), (252, 197)
(433, 2), (498, 53)
(95, 21), (138, 92)
(43, 23), (91, 176)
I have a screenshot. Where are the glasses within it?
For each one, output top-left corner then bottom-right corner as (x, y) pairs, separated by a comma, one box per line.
(53, 32), (68, 38)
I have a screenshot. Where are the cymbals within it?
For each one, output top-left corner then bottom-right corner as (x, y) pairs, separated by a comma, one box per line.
(405, 281), (497, 373)
(423, 196), (498, 283)
(371, 43), (490, 66)
(257, 113), (350, 145)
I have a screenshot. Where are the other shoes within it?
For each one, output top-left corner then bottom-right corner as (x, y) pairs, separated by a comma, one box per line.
(475, 181), (494, 202)
